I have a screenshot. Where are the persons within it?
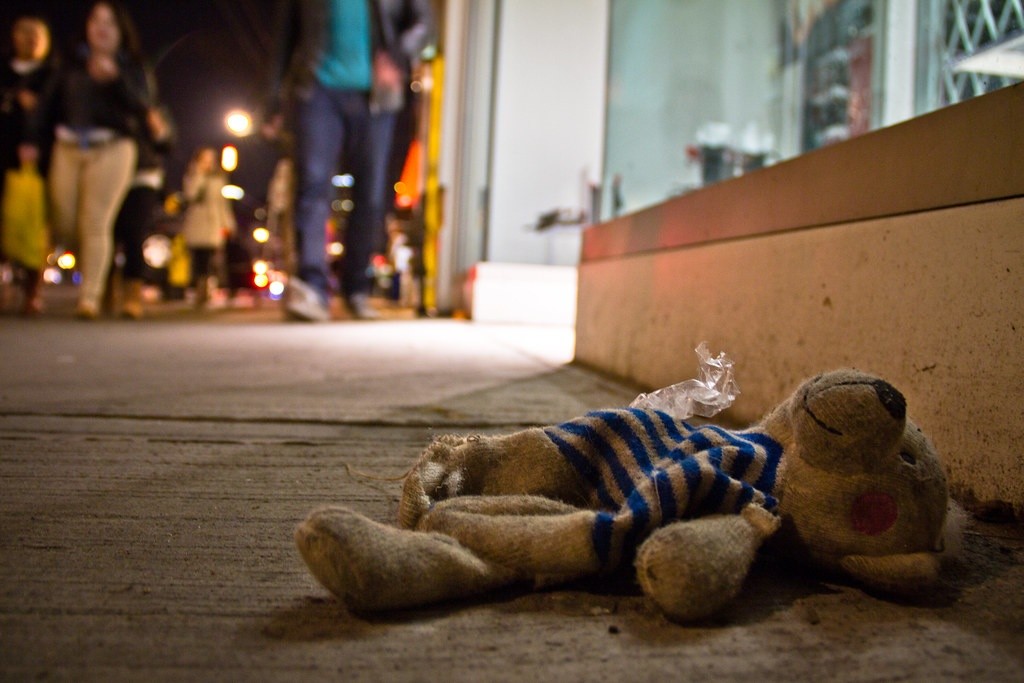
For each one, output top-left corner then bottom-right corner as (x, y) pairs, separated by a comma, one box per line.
(0, 1), (434, 320)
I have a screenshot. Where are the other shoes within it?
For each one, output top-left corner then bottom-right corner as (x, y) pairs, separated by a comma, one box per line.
(75, 300), (99, 317)
(285, 288), (330, 322)
(348, 294), (378, 319)
(123, 298), (146, 319)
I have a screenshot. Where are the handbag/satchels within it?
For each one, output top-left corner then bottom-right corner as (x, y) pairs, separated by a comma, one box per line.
(2, 153), (49, 269)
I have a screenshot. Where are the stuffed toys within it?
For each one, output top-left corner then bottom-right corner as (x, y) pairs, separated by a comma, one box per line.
(292, 367), (1023, 626)
